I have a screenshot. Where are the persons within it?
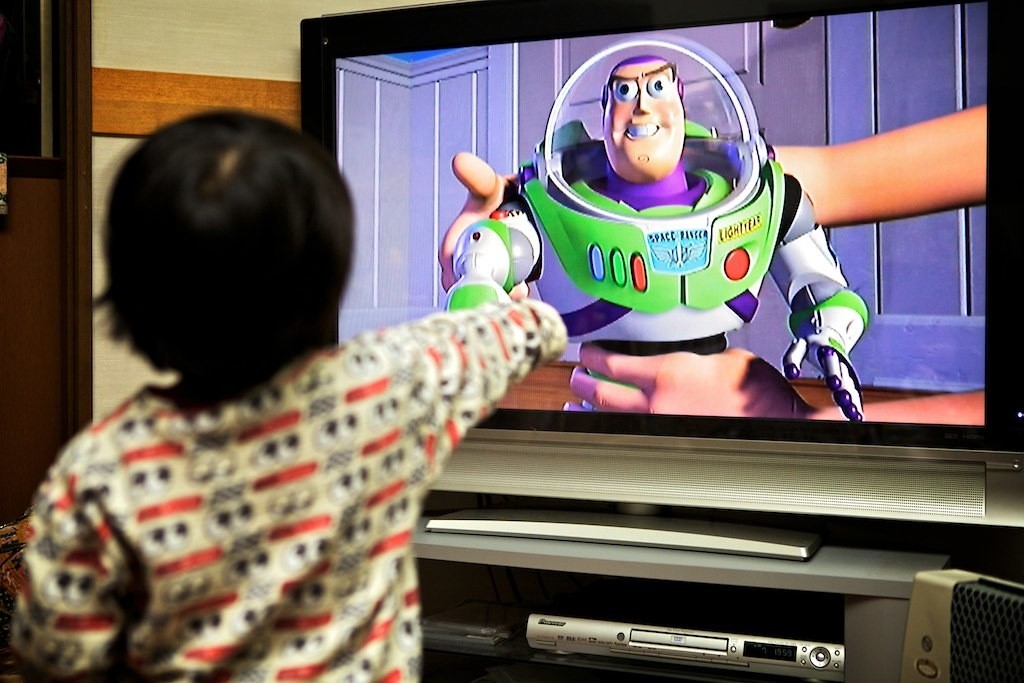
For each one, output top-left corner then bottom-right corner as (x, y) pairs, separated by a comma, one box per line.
(432, 104), (985, 424)
(445, 56), (871, 422)
(11, 109), (568, 683)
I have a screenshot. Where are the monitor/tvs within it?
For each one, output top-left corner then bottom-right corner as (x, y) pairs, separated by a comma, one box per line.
(299, 1), (1024, 528)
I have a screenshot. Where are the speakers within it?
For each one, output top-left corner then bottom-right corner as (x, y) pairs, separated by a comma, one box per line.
(901, 568), (1024, 683)
